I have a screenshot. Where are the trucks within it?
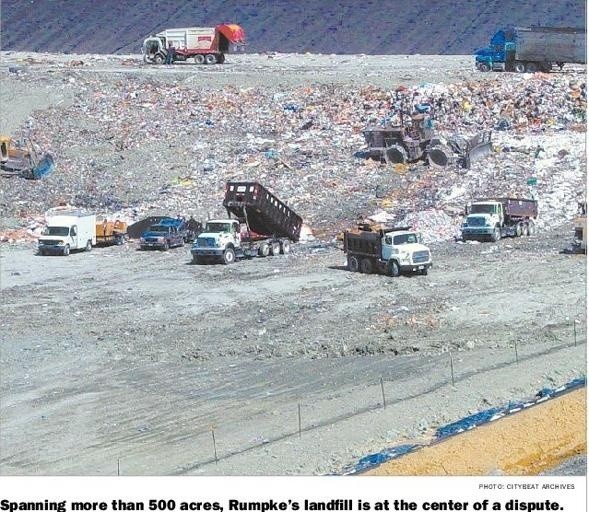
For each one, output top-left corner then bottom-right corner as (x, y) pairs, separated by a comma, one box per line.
(343, 225), (433, 279)
(475, 25), (587, 72)
(190, 180), (304, 265)
(38, 214), (97, 256)
(460, 196), (539, 240)
(142, 23), (244, 64)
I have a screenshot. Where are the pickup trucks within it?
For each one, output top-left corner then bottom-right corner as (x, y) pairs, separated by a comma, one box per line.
(137, 225), (186, 251)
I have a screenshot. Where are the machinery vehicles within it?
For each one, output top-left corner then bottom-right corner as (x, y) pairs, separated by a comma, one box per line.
(0, 133), (54, 180)
(574, 201), (587, 251)
(361, 102), (493, 168)
